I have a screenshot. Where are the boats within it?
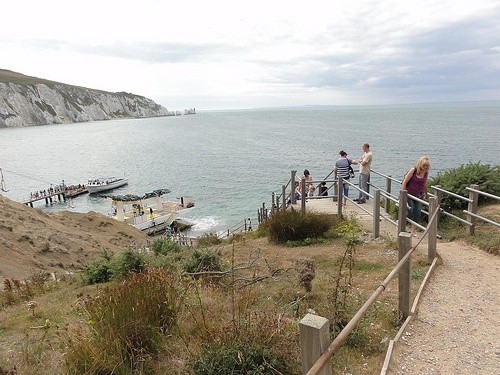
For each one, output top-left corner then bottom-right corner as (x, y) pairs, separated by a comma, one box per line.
(109, 194), (196, 235)
(86, 175), (128, 194)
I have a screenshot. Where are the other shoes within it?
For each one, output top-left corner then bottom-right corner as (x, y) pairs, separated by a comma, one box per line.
(357, 200), (366, 204)
(353, 198), (359, 201)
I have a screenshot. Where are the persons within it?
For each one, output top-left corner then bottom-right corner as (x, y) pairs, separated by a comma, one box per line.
(29, 169), (329, 245)
(334, 150), (358, 206)
(352, 143), (372, 204)
(401, 157), (430, 238)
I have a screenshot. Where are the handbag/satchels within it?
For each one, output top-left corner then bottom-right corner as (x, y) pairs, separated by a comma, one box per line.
(346, 158), (354, 178)
